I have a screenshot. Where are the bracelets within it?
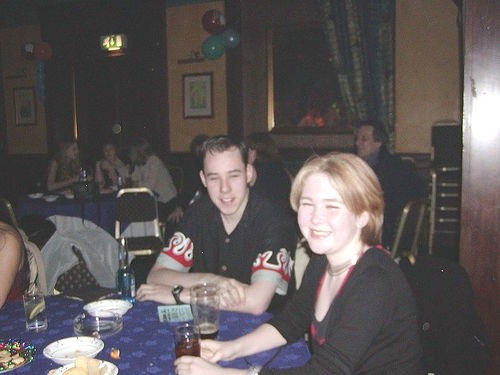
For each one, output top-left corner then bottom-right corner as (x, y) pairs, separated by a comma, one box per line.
(246, 363), (263, 375)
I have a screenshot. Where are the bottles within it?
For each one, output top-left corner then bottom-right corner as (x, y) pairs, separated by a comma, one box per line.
(115, 237), (136, 302)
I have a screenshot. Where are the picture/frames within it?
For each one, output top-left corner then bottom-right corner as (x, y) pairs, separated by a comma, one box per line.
(12, 87), (38, 126)
(182, 72), (213, 119)
(266, 24), (354, 134)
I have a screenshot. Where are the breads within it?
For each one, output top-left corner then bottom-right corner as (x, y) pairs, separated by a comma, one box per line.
(62, 356), (102, 375)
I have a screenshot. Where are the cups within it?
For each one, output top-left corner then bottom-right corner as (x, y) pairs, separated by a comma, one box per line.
(173, 324), (200, 358)
(190, 283), (221, 340)
(23, 291), (48, 333)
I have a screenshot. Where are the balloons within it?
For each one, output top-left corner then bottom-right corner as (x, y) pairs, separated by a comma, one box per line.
(22, 40), (52, 64)
(201, 36), (224, 59)
(223, 29), (240, 49)
(202, 10), (227, 35)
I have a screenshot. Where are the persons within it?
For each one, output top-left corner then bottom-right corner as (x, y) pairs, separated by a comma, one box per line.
(355, 119), (411, 252)
(40, 136), (180, 246)
(173, 151), (426, 375)
(134, 134), (298, 321)
(0, 221), (33, 314)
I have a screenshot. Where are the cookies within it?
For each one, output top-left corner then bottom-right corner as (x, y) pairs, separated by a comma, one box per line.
(0, 350), (24, 368)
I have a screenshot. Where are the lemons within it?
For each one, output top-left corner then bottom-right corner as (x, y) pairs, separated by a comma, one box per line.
(29, 302), (45, 319)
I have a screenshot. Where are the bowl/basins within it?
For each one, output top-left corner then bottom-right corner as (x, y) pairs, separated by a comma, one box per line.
(27, 193), (76, 202)
(53, 359), (119, 374)
(43, 336), (104, 365)
(82, 299), (134, 317)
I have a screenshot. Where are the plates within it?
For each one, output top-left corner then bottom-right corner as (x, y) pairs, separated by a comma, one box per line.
(0, 338), (37, 374)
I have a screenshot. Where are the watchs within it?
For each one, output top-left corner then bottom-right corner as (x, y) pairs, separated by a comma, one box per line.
(172, 285), (187, 304)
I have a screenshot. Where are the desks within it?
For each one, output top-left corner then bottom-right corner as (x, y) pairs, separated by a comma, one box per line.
(17, 186), (159, 235)
(0, 285), (312, 375)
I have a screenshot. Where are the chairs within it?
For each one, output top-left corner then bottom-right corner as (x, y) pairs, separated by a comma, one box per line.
(114, 188), (163, 256)
(0, 196), (119, 296)
(392, 119), (462, 258)
